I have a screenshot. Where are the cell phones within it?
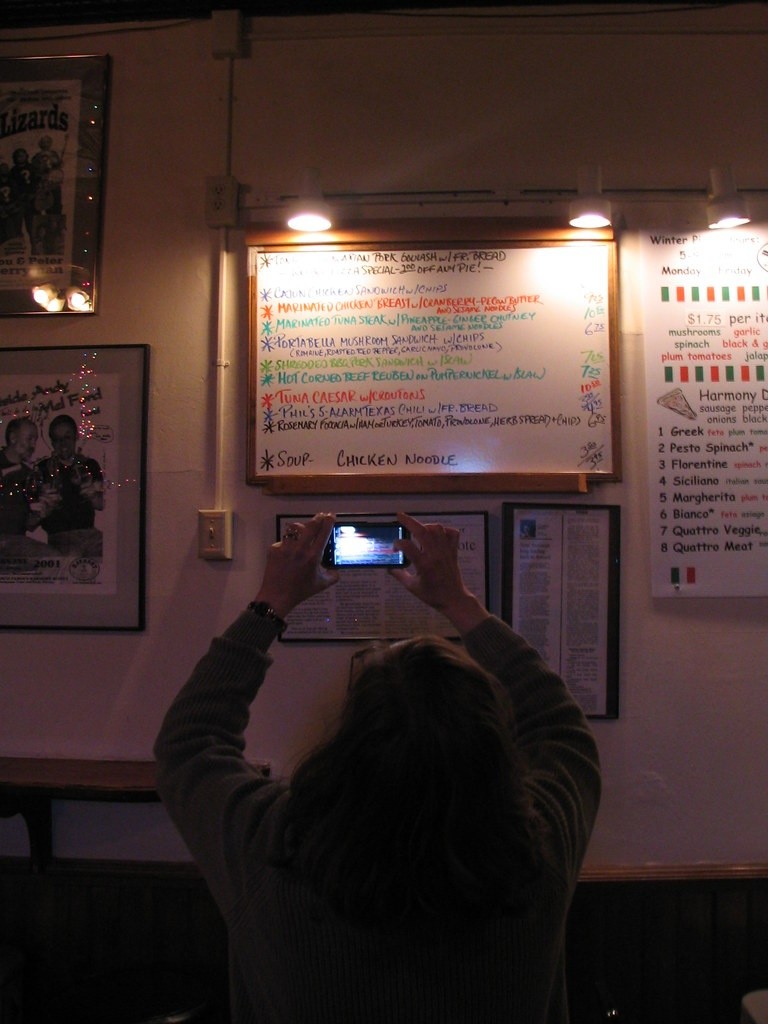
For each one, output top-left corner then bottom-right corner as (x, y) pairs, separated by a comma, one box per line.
(323, 522), (411, 568)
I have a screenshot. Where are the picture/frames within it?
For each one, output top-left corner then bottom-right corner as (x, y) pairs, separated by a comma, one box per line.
(1, 53), (113, 316)
(0, 342), (151, 630)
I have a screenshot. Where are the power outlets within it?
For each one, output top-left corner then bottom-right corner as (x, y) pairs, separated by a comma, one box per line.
(203, 174), (239, 226)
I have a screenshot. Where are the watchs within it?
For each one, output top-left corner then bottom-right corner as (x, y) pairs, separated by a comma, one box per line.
(246, 601), (288, 634)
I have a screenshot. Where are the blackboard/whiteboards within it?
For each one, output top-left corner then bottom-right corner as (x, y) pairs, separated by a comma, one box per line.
(243, 215), (624, 493)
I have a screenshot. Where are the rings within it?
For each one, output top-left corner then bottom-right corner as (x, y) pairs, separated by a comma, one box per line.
(283, 530), (299, 539)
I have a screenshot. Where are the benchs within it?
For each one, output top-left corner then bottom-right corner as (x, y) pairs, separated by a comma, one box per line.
(0, 754), (272, 872)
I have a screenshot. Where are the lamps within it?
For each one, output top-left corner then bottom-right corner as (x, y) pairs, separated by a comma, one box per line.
(280, 157), (753, 233)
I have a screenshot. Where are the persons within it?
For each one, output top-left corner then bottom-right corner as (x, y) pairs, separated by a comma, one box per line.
(0, 418), (63, 568)
(520, 524), (533, 537)
(0, 135), (66, 256)
(36, 415), (104, 557)
(150, 509), (602, 1024)
